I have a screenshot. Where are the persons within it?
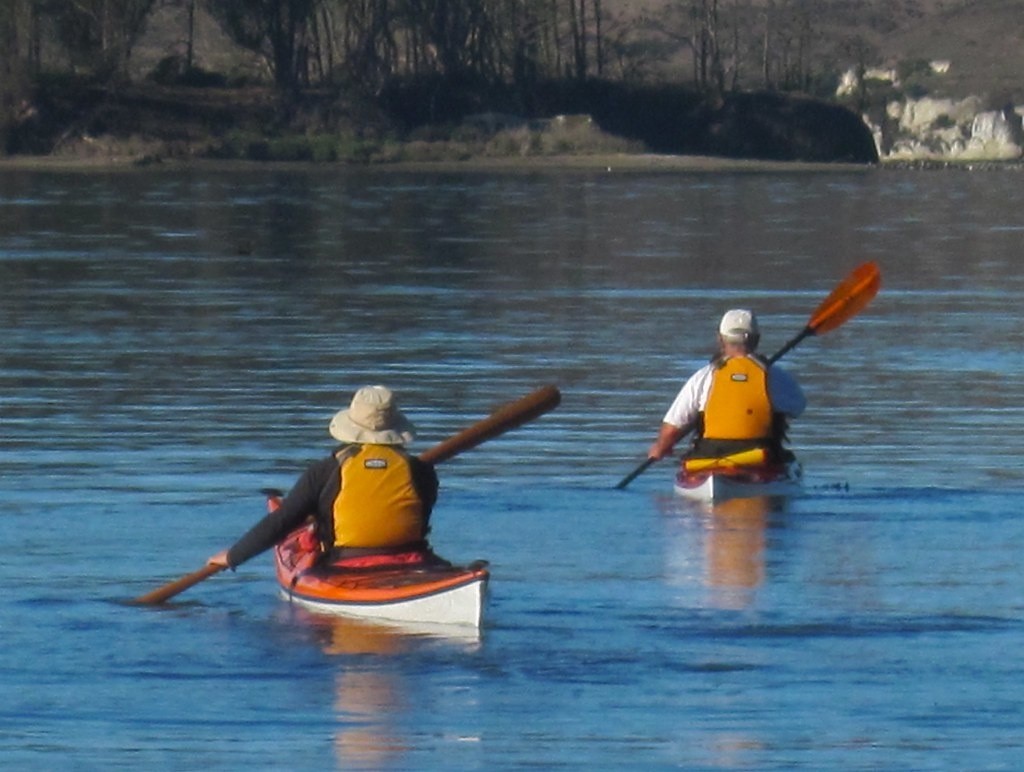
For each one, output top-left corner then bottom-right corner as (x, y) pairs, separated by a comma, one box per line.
(649, 309), (807, 463)
(207, 386), (439, 572)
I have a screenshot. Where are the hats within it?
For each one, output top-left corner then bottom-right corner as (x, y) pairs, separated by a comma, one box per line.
(719, 309), (762, 340)
(329, 386), (417, 445)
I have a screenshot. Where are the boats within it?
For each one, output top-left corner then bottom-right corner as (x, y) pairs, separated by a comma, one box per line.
(265, 489), (492, 643)
(674, 452), (803, 510)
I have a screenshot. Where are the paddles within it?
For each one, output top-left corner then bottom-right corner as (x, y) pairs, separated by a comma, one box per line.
(128, 382), (565, 606)
(611, 260), (883, 491)
